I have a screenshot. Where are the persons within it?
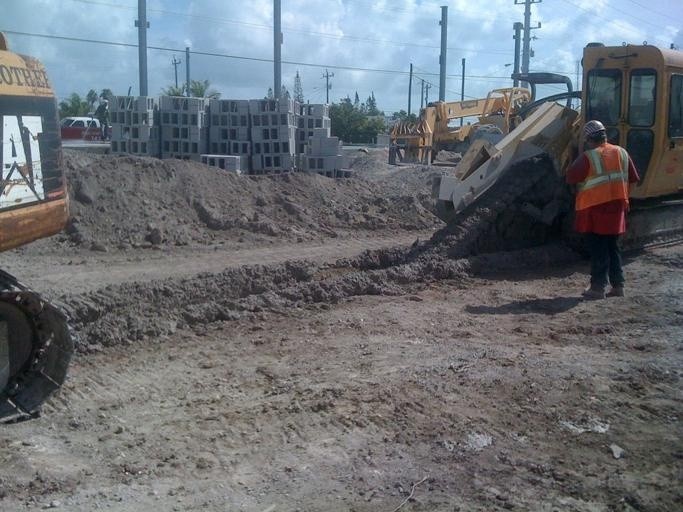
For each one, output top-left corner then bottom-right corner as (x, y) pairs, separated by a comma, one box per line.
(564, 120), (640, 300)
(94, 100), (109, 141)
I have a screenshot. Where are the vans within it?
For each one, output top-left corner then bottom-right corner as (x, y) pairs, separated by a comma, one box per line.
(61, 116), (101, 129)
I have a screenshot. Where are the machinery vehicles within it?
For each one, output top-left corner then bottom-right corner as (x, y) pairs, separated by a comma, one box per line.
(0, 32), (75, 426)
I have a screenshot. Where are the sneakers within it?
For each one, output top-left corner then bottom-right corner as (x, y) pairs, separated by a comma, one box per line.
(582, 288), (604, 299)
(605, 286), (623, 297)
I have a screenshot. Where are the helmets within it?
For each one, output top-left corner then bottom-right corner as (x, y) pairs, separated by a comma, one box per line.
(582, 120), (606, 139)
